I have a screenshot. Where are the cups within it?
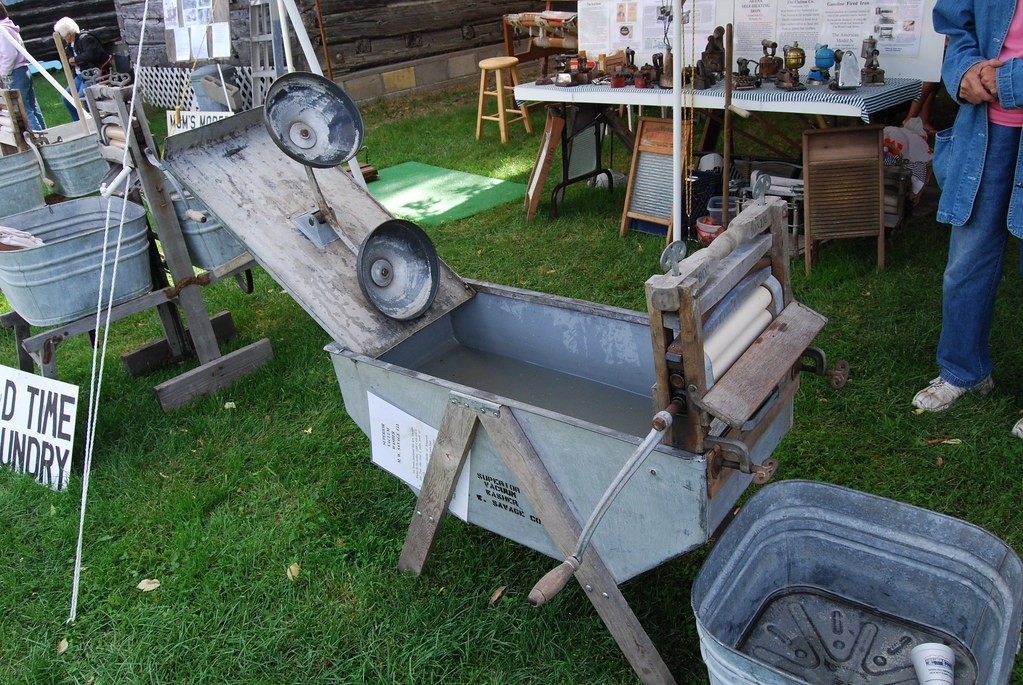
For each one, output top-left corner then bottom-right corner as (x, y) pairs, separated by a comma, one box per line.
(910, 642), (955, 685)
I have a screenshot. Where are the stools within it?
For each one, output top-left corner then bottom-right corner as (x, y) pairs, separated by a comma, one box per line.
(475, 57), (534, 145)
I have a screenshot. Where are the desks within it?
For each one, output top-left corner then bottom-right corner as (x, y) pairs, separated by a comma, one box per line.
(513, 77), (924, 221)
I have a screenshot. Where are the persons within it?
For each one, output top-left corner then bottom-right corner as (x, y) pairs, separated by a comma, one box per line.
(912, 0), (1023, 439)
(54, 16), (113, 122)
(902, 82), (942, 135)
(0, 2), (46, 131)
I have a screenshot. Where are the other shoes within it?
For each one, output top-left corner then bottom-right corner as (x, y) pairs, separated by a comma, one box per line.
(911, 371), (997, 413)
(1011, 418), (1023, 439)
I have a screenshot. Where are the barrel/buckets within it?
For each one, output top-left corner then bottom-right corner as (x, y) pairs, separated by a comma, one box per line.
(0, 147), (46, 216)
(33, 118), (110, 198)
(165, 178), (246, 270)
(696, 216), (722, 244)
(0, 195), (153, 327)
(691, 479), (1023, 685)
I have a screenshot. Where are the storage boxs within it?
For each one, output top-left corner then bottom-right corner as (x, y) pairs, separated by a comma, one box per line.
(628, 169), (722, 236)
(707, 195), (736, 226)
